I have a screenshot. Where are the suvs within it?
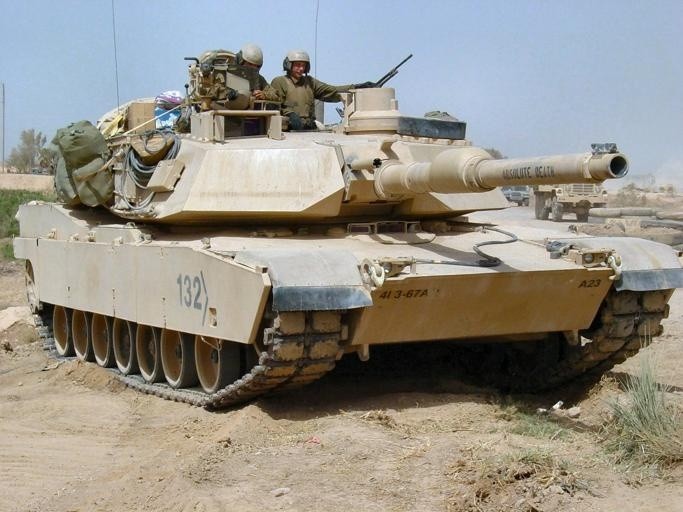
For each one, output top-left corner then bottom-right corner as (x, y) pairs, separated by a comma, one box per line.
(501, 185), (530, 206)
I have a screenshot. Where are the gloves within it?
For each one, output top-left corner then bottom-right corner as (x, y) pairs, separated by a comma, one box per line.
(355, 82), (377, 88)
(286, 112), (301, 130)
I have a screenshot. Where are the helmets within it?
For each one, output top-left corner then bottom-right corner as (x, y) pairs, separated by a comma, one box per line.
(236, 46), (263, 70)
(283, 50), (310, 73)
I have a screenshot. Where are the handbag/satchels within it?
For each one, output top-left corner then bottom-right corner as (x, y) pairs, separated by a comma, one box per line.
(50, 121), (114, 208)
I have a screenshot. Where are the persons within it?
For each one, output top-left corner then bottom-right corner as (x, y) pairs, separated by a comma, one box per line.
(270, 49), (380, 130)
(235, 45), (271, 100)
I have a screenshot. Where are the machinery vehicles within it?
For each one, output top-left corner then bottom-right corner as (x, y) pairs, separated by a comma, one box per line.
(535, 184), (608, 220)
(13, 83), (683, 411)
